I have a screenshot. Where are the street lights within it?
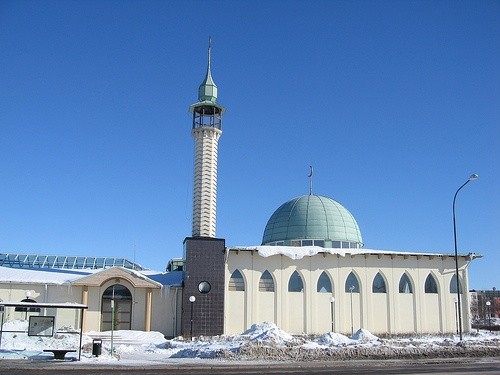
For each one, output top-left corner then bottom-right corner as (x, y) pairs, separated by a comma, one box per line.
(189, 295), (196, 340)
(329, 296), (335, 332)
(453, 174), (479, 340)
(24, 290), (31, 320)
(486, 300), (491, 332)
(349, 285), (355, 337)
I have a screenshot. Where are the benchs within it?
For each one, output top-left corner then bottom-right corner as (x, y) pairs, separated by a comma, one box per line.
(43, 350), (77, 359)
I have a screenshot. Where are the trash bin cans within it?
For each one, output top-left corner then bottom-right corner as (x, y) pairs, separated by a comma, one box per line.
(93, 338), (102, 357)
